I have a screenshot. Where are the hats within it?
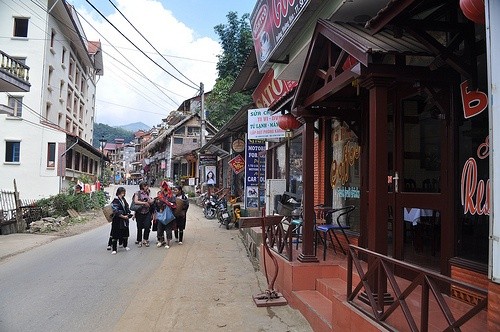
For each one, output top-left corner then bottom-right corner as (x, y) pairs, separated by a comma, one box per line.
(160, 182), (169, 190)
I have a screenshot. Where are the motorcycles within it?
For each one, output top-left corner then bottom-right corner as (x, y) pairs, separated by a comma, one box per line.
(216, 200), (232, 230)
(199, 192), (219, 220)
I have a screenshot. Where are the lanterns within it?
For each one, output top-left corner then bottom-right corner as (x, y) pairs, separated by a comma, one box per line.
(459, 0), (485, 24)
(279, 113), (302, 148)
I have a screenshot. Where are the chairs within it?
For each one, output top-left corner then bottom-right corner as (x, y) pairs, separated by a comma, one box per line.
(281, 205), (356, 261)
(387, 178), (442, 256)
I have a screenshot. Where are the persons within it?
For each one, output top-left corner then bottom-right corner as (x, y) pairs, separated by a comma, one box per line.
(207, 171), (214, 183)
(107, 187), (131, 254)
(150, 191), (176, 248)
(134, 181), (154, 247)
(172, 186), (189, 244)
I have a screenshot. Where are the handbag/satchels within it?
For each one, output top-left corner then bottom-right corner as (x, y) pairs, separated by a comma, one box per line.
(130, 191), (142, 211)
(156, 205), (175, 226)
(102, 203), (115, 222)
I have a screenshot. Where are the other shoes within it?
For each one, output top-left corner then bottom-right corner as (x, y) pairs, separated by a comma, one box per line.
(125, 247), (130, 251)
(146, 240), (149, 246)
(137, 244), (142, 248)
(119, 245), (123, 248)
(112, 251), (117, 254)
(175, 238), (178, 242)
(143, 240), (147, 244)
(135, 241), (139, 244)
(107, 246), (111, 250)
(178, 242), (182, 244)
(157, 242), (162, 247)
(165, 245), (170, 249)
(156, 241), (159, 243)
(162, 241), (166, 244)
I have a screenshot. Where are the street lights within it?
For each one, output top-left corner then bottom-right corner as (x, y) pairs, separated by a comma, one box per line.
(99, 135), (108, 191)
